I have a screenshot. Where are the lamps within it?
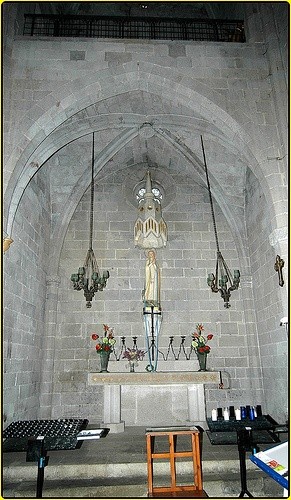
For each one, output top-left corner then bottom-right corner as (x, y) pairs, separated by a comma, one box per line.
(200, 134), (241, 308)
(70, 131), (109, 308)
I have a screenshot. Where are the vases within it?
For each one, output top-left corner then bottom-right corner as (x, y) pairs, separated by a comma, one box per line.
(129, 359), (136, 371)
(198, 352), (209, 372)
(97, 351), (112, 372)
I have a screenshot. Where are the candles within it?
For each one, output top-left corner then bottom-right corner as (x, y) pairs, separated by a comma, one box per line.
(211, 405), (262, 422)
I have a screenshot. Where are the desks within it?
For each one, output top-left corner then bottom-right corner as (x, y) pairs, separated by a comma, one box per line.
(144, 426), (208, 497)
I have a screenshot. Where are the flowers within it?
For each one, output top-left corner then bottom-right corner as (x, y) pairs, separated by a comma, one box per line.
(93, 324), (116, 353)
(191, 324), (214, 355)
(121, 348), (145, 367)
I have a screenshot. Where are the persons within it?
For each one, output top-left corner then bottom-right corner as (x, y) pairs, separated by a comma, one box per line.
(144, 251), (160, 310)
